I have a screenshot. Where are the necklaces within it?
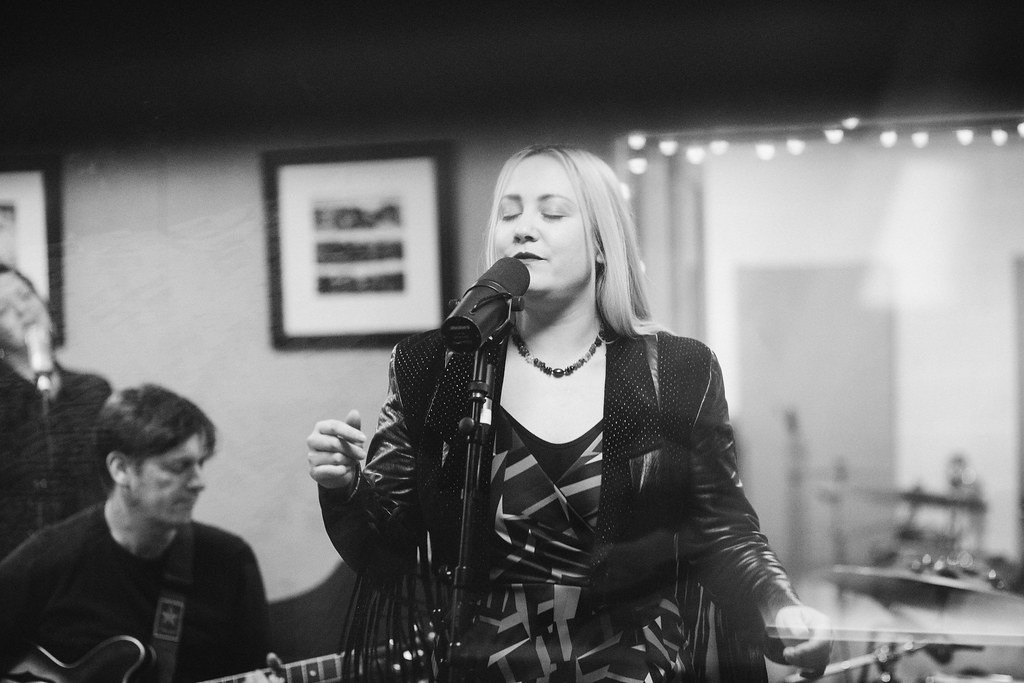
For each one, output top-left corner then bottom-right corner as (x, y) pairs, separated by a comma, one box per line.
(509, 326), (605, 378)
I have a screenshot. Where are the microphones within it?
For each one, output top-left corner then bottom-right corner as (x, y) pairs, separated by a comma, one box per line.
(440, 257), (531, 355)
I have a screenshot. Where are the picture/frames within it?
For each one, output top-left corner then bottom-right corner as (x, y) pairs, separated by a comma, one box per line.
(260, 135), (454, 353)
(0, 153), (65, 348)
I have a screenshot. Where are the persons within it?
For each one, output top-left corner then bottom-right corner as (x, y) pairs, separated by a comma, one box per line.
(306, 142), (834, 683)
(1, 381), (288, 683)
(0, 261), (115, 565)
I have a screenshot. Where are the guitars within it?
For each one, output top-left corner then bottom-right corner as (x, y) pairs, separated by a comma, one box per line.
(4, 617), (444, 683)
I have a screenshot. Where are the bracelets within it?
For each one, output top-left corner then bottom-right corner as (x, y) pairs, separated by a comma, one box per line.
(348, 466), (362, 499)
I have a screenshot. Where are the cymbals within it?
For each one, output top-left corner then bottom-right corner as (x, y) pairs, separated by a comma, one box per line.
(828, 558), (1022, 612)
